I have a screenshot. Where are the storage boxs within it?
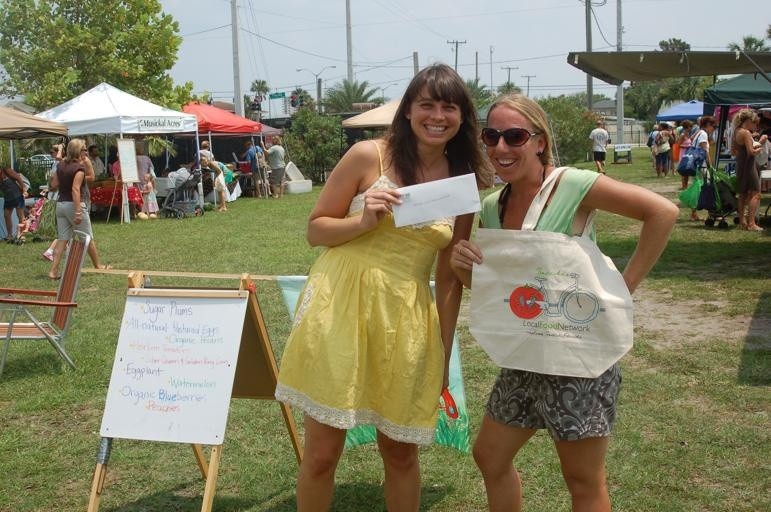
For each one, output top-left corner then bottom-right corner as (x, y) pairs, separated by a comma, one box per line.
(286, 180), (312, 193)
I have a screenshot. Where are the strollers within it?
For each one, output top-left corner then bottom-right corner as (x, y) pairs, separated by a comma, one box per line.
(9, 186), (58, 245)
(157, 168), (213, 219)
(695, 162), (742, 230)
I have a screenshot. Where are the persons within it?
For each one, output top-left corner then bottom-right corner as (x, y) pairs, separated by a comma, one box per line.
(588, 119), (610, 175)
(195, 132), (286, 211)
(275, 63), (496, 511)
(0, 137), (157, 280)
(647, 109), (771, 232)
(449, 94), (679, 512)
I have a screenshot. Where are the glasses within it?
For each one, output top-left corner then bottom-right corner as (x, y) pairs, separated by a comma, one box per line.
(480, 126), (544, 149)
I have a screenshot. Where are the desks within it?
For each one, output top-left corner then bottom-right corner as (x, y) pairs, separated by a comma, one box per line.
(240, 171), (272, 198)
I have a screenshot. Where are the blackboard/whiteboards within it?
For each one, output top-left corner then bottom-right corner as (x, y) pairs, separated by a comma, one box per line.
(99, 295), (248, 443)
(116, 138), (140, 183)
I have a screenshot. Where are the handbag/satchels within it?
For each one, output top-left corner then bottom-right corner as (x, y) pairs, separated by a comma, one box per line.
(646, 131), (654, 147)
(655, 131), (667, 145)
(469, 165), (640, 380)
(675, 130), (708, 177)
(677, 166), (739, 216)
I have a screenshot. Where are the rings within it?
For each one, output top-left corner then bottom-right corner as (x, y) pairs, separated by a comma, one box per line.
(458, 248), (462, 253)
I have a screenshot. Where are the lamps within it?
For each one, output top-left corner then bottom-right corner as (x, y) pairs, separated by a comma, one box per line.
(573, 54), (578, 65)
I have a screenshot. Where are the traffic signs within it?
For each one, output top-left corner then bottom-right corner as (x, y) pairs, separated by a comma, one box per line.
(269, 92), (285, 100)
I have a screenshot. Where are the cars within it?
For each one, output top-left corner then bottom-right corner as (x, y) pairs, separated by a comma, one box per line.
(25, 153), (55, 169)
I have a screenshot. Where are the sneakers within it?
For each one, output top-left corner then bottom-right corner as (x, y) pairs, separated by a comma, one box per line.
(739, 223), (764, 232)
(42, 251), (54, 263)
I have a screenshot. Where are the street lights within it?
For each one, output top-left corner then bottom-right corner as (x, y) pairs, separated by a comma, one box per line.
(295, 65), (337, 111)
(365, 82), (400, 105)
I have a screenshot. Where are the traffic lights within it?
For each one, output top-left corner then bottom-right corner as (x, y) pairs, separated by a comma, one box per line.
(290, 95), (297, 107)
(298, 94), (304, 107)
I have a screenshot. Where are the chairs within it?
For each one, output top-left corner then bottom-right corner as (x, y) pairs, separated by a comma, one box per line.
(0, 229), (92, 373)
